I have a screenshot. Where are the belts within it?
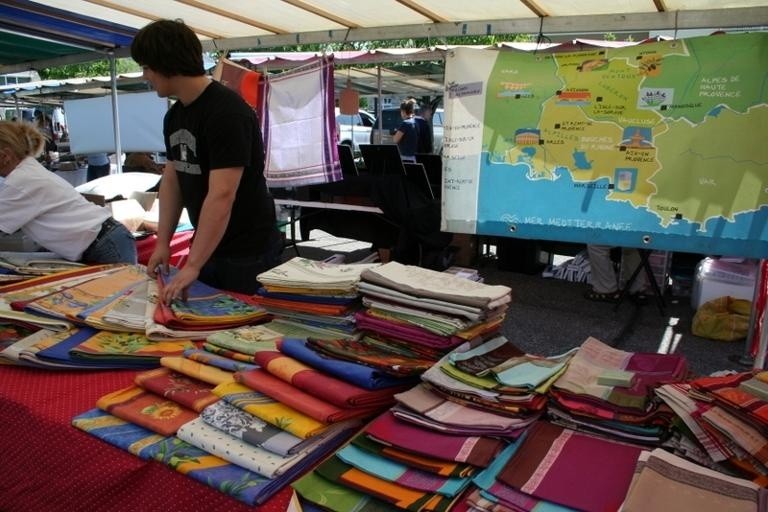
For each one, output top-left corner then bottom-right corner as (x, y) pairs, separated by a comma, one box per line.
(82, 216), (116, 257)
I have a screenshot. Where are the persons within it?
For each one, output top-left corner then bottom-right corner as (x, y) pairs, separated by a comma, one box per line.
(392, 98), (432, 155)
(584, 245), (648, 305)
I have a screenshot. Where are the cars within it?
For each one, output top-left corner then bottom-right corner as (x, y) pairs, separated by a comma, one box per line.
(334, 105), (444, 159)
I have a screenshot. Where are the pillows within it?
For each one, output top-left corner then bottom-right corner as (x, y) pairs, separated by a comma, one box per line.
(75, 171), (162, 202)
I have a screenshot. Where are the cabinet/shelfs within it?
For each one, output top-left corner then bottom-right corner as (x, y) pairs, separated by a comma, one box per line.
(619, 247), (673, 296)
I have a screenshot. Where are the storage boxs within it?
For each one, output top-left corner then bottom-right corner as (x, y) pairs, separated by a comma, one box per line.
(690, 257), (756, 313)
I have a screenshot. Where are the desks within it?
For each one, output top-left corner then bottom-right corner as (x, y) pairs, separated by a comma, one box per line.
(276, 197), (385, 258)
